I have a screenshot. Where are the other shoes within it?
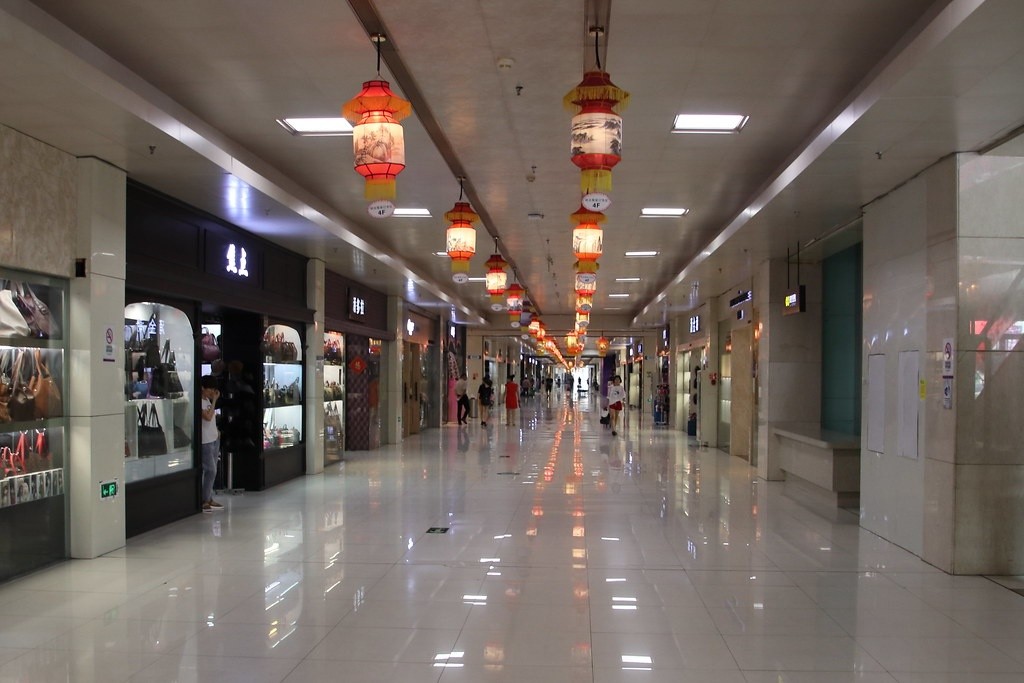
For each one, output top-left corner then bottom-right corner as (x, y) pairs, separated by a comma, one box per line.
(612, 431), (617, 436)
(203, 502), (213, 511)
(481, 421), (483, 425)
(459, 422), (461, 424)
(462, 418), (467, 424)
(483, 422), (487, 427)
(209, 499), (224, 508)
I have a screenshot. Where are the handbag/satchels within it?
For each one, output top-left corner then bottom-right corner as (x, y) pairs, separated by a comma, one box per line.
(125, 314), (192, 458)
(263, 331), (299, 445)
(0, 276), (62, 480)
(600, 411), (610, 424)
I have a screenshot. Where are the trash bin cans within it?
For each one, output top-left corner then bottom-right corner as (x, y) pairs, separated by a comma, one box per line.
(470, 398), (479, 418)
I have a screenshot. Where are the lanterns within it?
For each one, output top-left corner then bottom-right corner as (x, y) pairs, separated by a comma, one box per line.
(343, 69), (628, 370)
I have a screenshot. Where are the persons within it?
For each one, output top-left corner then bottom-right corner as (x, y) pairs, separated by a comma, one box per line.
(522, 377), (531, 397)
(200, 375), (225, 512)
(503, 374), (521, 426)
(545, 374), (553, 398)
(477, 374), (493, 428)
(607, 374), (625, 436)
(454, 373), (470, 425)
